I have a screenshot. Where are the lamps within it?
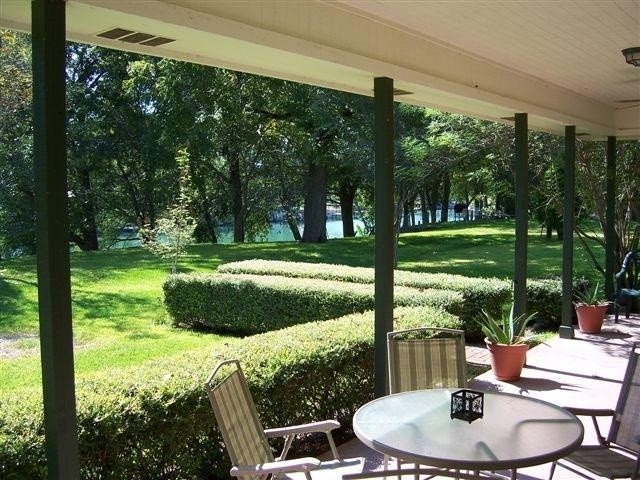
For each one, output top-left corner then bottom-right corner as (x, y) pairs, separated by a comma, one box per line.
(621, 46), (640, 69)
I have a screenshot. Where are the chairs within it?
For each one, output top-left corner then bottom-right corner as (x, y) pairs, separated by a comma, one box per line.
(385, 324), (469, 480)
(614, 249), (640, 322)
(547, 339), (640, 480)
(204, 357), (361, 479)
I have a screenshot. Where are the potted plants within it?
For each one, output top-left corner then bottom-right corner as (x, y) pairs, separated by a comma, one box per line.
(573, 279), (622, 335)
(472, 301), (552, 382)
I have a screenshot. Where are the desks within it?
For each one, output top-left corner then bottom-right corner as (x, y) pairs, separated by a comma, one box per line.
(351, 387), (586, 480)
